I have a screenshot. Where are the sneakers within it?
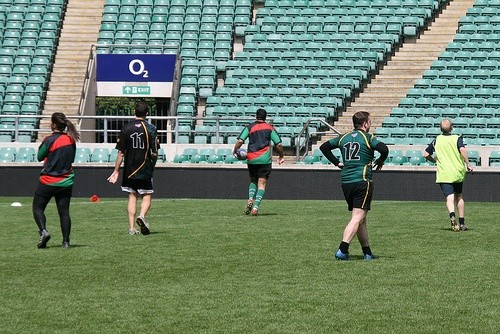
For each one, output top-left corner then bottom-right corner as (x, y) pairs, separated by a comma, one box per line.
(129, 230), (139, 236)
(37, 230), (50, 249)
(61, 241), (69, 247)
(460, 224), (467, 231)
(245, 200), (253, 214)
(335, 249), (350, 260)
(136, 216), (149, 235)
(450, 216), (459, 231)
(251, 208), (258, 215)
(363, 254), (374, 260)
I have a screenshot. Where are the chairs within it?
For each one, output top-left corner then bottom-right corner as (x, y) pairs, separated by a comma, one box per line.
(0, 0), (500, 167)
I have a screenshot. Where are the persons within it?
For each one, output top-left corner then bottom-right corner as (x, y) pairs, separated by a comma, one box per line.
(319, 111), (389, 260)
(113, 102), (160, 236)
(233, 109), (284, 215)
(32, 112), (81, 249)
(424, 119), (473, 232)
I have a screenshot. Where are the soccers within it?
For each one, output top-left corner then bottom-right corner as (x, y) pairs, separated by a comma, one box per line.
(236, 148), (247, 161)
